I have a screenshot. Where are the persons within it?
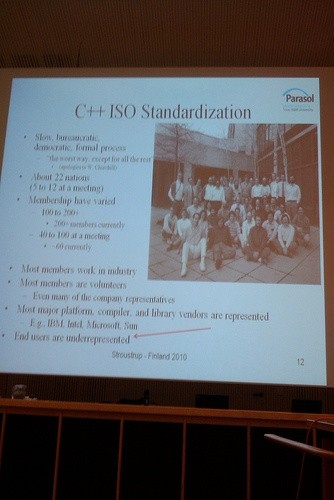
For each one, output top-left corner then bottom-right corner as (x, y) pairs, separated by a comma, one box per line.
(161, 162), (310, 278)
(9, 384), (37, 400)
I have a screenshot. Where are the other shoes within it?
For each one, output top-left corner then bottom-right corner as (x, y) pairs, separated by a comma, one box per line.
(200, 262), (206, 272)
(180, 265), (189, 277)
(216, 259), (221, 270)
(261, 259), (268, 266)
